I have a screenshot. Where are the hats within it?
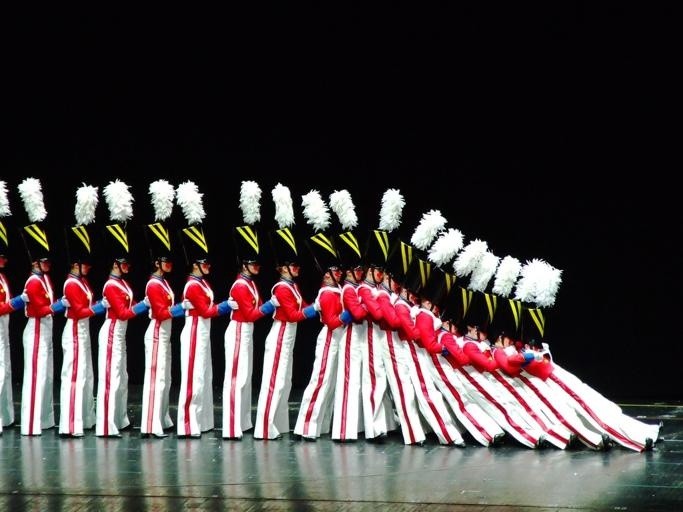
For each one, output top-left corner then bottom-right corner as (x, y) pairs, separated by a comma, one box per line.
(1, 181), (11, 257)
(65, 182), (100, 264)
(300, 190), (341, 274)
(236, 180), (263, 264)
(384, 209), (563, 349)
(329, 191), (364, 267)
(366, 189), (407, 269)
(145, 179), (177, 261)
(105, 179), (134, 262)
(270, 182), (302, 267)
(18, 178), (53, 265)
(178, 182), (208, 264)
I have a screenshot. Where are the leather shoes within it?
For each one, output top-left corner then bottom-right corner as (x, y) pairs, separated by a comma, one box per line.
(140, 432), (169, 440)
(59, 432), (86, 440)
(488, 430), (655, 453)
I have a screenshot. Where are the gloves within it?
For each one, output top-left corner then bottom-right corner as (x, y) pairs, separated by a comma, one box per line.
(141, 295), (151, 309)
(267, 294), (281, 310)
(59, 294), (71, 310)
(18, 288), (31, 304)
(311, 297), (322, 312)
(100, 295), (111, 310)
(532, 350), (544, 363)
(225, 295), (239, 312)
(180, 296), (196, 311)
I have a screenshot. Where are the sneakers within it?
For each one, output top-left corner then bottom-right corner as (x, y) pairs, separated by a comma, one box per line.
(220, 434), (468, 447)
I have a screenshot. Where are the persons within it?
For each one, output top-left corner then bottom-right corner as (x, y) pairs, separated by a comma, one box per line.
(57, 253), (109, 439)
(293, 257), (664, 451)
(220, 258), (281, 438)
(93, 255), (151, 439)
(138, 253), (195, 439)
(176, 251), (240, 439)
(0, 246), (29, 439)
(20, 257), (71, 435)
(252, 261), (321, 441)
